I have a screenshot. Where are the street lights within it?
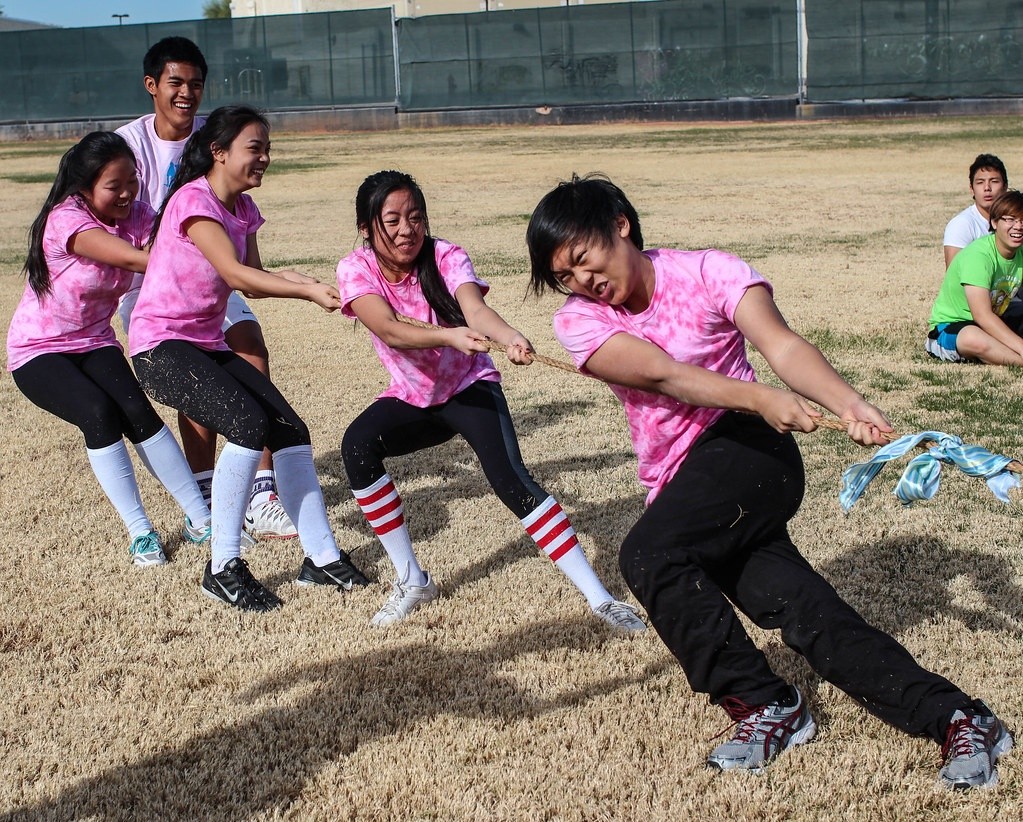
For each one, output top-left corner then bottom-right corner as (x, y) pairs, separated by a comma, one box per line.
(112, 14), (130, 26)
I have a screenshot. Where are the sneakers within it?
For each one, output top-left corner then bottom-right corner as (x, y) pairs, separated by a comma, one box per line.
(199, 556), (285, 614)
(705, 683), (818, 775)
(128, 530), (169, 569)
(367, 560), (439, 626)
(591, 600), (646, 631)
(935, 698), (1017, 792)
(296, 545), (371, 594)
(242, 493), (299, 541)
(181, 515), (257, 549)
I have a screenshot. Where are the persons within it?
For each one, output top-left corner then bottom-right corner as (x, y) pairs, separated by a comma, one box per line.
(342, 170), (644, 630)
(5, 36), (298, 567)
(128, 107), (370, 614)
(944, 154), (1022, 334)
(529, 175), (1010, 789)
(927, 190), (1023, 364)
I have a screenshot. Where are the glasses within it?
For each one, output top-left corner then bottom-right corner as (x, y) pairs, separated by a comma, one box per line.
(998, 216), (1023, 224)
